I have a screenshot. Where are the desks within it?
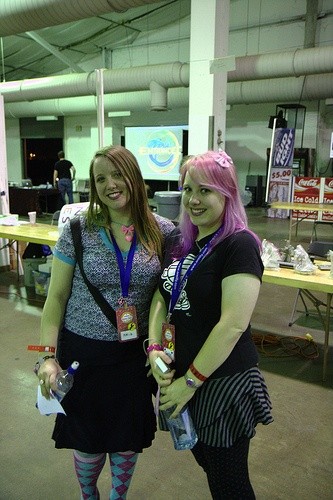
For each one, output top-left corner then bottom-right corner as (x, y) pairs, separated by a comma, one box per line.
(9, 187), (60, 215)
(270, 202), (333, 245)
(0, 221), (60, 281)
(261, 256), (333, 383)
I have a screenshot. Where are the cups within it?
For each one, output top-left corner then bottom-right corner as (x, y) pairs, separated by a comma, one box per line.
(28, 212), (36, 224)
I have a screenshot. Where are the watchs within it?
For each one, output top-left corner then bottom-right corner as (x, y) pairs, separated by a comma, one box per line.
(184, 372), (202, 388)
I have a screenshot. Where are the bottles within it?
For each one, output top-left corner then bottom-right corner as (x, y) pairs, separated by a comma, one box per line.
(36, 362), (80, 416)
(162, 402), (198, 450)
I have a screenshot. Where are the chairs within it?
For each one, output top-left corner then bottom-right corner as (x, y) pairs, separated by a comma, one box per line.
(309, 212), (333, 243)
(288, 244), (333, 332)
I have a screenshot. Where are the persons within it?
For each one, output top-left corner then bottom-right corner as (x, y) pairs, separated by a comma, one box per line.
(53, 151), (76, 204)
(28, 144), (175, 500)
(147, 148), (273, 500)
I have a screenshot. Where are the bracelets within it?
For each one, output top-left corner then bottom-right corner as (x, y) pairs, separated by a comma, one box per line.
(34, 355), (55, 374)
(147, 344), (163, 352)
(28, 345), (55, 353)
(189, 363), (207, 381)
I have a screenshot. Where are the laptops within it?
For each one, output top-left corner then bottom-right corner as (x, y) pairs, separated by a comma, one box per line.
(22, 179), (33, 187)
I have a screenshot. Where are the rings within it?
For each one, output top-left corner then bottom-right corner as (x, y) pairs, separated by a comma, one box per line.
(39, 379), (44, 385)
